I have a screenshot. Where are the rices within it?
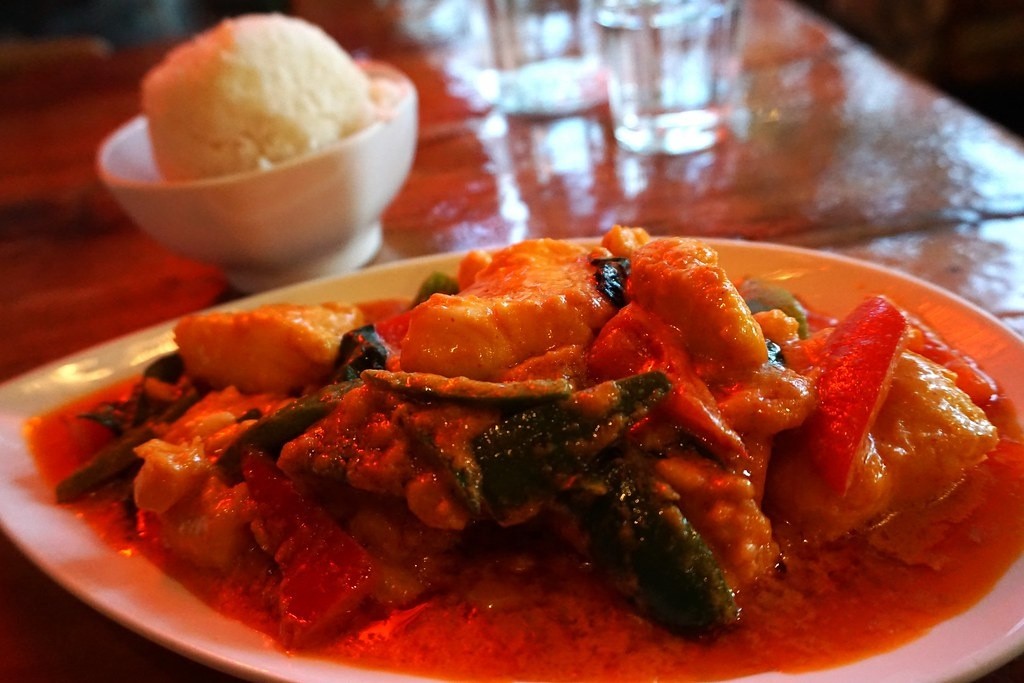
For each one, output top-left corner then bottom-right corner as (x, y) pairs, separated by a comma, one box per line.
(143, 14), (381, 180)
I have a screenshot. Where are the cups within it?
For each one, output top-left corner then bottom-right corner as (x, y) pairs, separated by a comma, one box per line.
(480, 1), (610, 115)
(592, 2), (744, 155)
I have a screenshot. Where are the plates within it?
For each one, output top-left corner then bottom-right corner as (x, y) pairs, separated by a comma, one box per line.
(0, 236), (1023, 682)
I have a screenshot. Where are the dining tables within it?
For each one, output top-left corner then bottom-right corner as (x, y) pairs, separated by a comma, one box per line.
(0, 4), (1022, 683)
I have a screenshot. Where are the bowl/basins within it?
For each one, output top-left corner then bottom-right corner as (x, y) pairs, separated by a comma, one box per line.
(96, 58), (418, 297)
